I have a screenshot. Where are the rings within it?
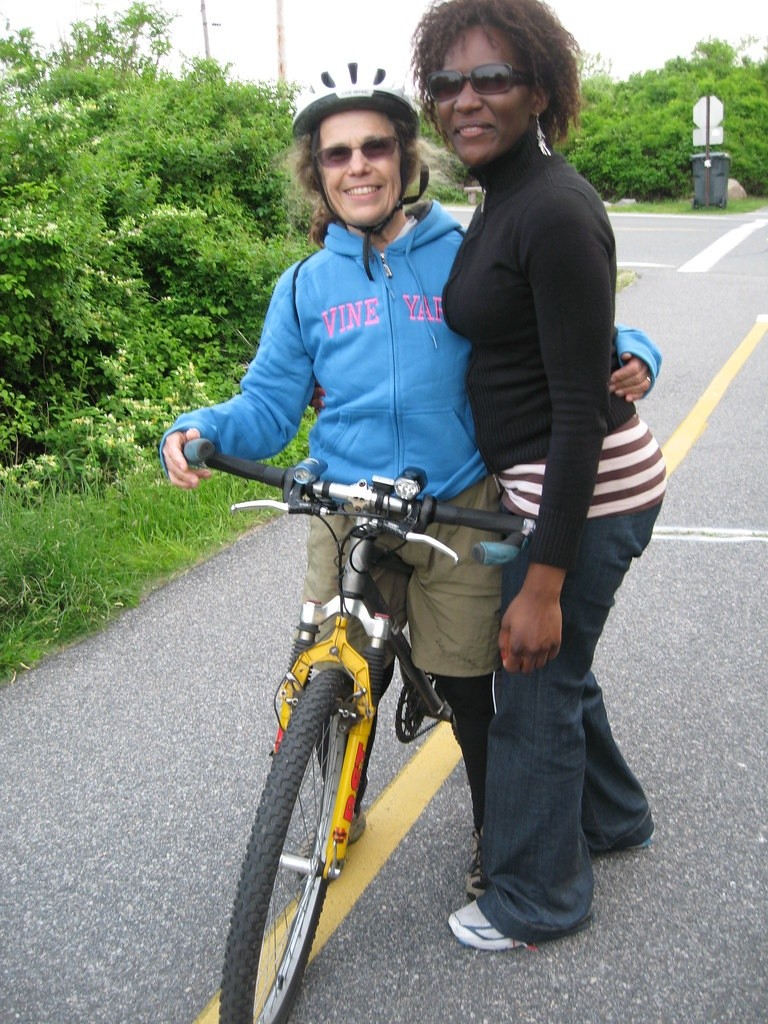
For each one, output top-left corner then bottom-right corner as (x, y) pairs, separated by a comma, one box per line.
(646, 377), (652, 383)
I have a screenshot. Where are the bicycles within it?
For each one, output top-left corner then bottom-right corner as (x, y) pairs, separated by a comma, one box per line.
(179, 440), (536, 1024)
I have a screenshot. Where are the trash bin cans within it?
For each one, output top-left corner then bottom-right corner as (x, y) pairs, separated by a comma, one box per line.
(690, 153), (732, 210)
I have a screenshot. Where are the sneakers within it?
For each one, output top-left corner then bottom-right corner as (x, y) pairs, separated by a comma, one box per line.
(465, 825), (498, 904)
(447, 901), (541, 953)
(345, 801), (367, 843)
(626, 825), (655, 849)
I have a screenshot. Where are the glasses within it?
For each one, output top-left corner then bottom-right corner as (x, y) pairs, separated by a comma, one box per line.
(426, 63), (534, 102)
(313, 137), (403, 168)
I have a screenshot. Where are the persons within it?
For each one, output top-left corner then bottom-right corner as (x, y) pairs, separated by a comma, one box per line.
(406, 1), (670, 951)
(159, 61), (662, 914)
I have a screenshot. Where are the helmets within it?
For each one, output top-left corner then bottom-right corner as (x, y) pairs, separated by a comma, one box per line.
(292, 57), (420, 141)
